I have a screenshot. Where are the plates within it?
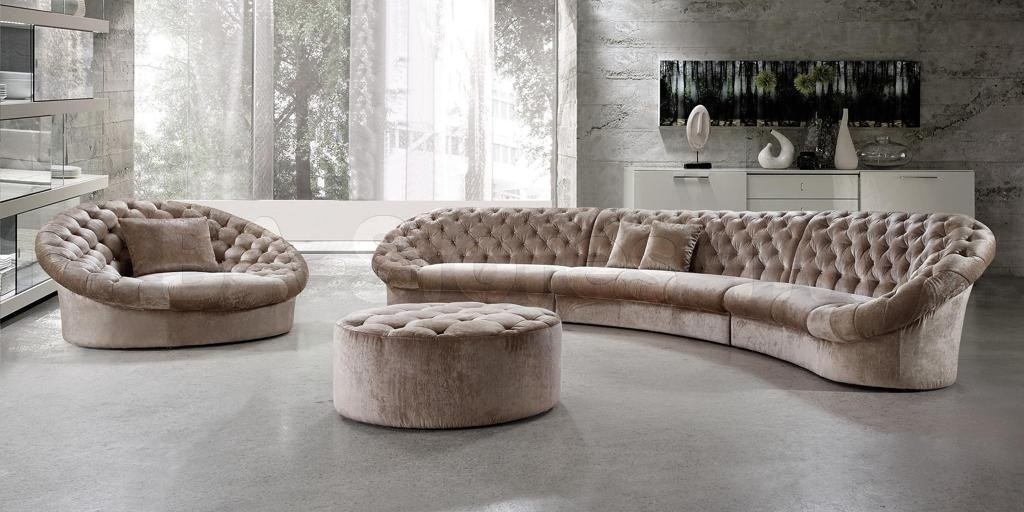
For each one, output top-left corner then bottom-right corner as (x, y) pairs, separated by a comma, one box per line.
(51, 164), (82, 179)
(0, 251), (32, 270)
(0, 84), (7, 101)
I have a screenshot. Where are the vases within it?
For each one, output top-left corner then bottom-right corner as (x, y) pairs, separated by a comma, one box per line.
(834, 108), (859, 170)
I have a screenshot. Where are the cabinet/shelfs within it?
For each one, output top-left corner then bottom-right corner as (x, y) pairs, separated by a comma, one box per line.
(0, 2), (111, 321)
(623, 167), (746, 211)
(859, 171), (976, 219)
(746, 171), (859, 214)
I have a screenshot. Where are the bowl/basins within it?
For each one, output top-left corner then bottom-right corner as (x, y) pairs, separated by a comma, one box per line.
(0, 71), (34, 99)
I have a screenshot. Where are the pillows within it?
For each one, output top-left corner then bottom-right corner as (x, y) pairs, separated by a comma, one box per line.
(118, 216), (220, 277)
(639, 220), (703, 272)
(604, 219), (651, 268)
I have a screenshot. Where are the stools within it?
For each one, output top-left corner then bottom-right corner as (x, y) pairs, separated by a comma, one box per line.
(333, 302), (561, 429)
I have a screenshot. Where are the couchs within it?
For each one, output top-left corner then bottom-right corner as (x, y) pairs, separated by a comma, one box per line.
(371, 207), (997, 392)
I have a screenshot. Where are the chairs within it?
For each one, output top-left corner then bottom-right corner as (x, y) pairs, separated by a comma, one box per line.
(35, 198), (308, 350)
